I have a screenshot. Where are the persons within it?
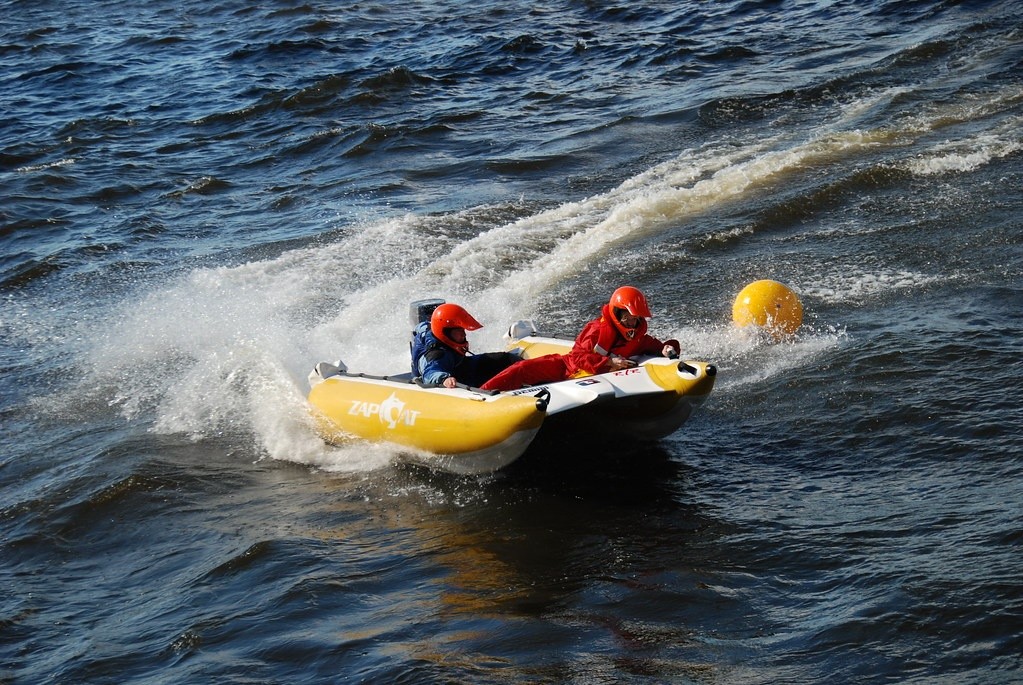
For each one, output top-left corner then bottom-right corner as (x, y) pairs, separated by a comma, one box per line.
(479, 286), (680, 390)
(411, 303), (525, 388)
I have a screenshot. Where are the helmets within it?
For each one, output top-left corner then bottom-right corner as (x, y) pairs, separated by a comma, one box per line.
(609, 285), (653, 343)
(430, 303), (484, 356)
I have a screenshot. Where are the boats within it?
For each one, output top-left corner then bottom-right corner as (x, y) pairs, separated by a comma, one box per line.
(309, 319), (720, 475)
